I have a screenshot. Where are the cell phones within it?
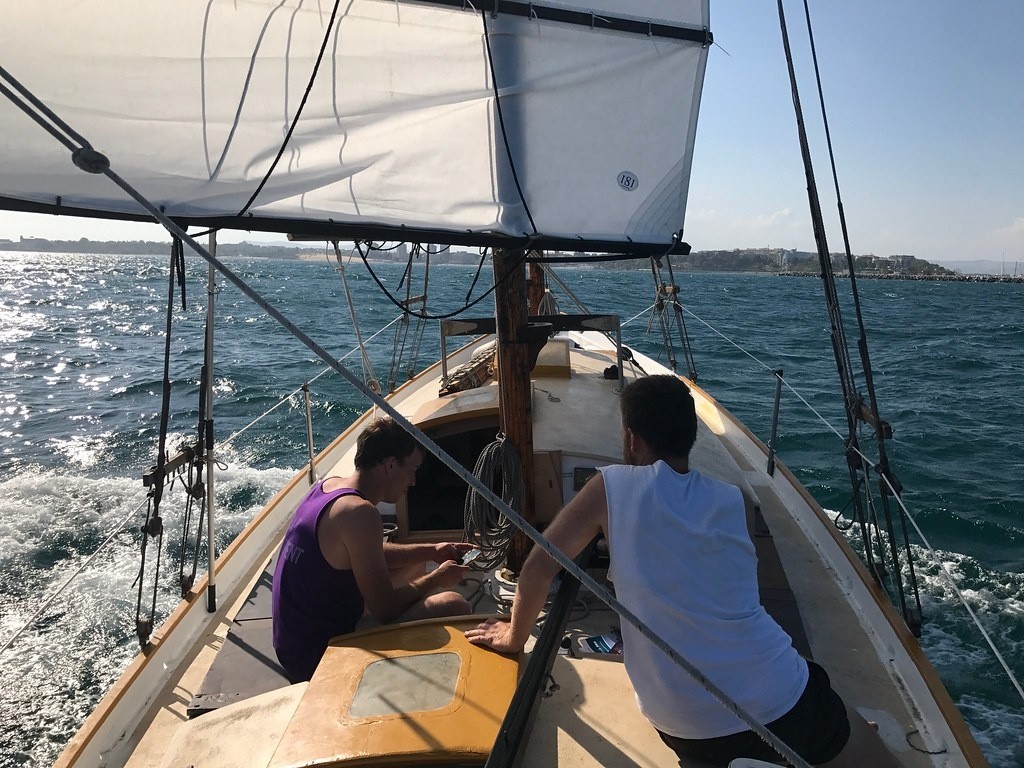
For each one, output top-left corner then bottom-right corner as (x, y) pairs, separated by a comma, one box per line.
(456, 548), (482, 567)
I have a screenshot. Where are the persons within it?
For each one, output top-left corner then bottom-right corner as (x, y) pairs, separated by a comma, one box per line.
(465, 374), (905, 768)
(271, 418), (478, 682)
